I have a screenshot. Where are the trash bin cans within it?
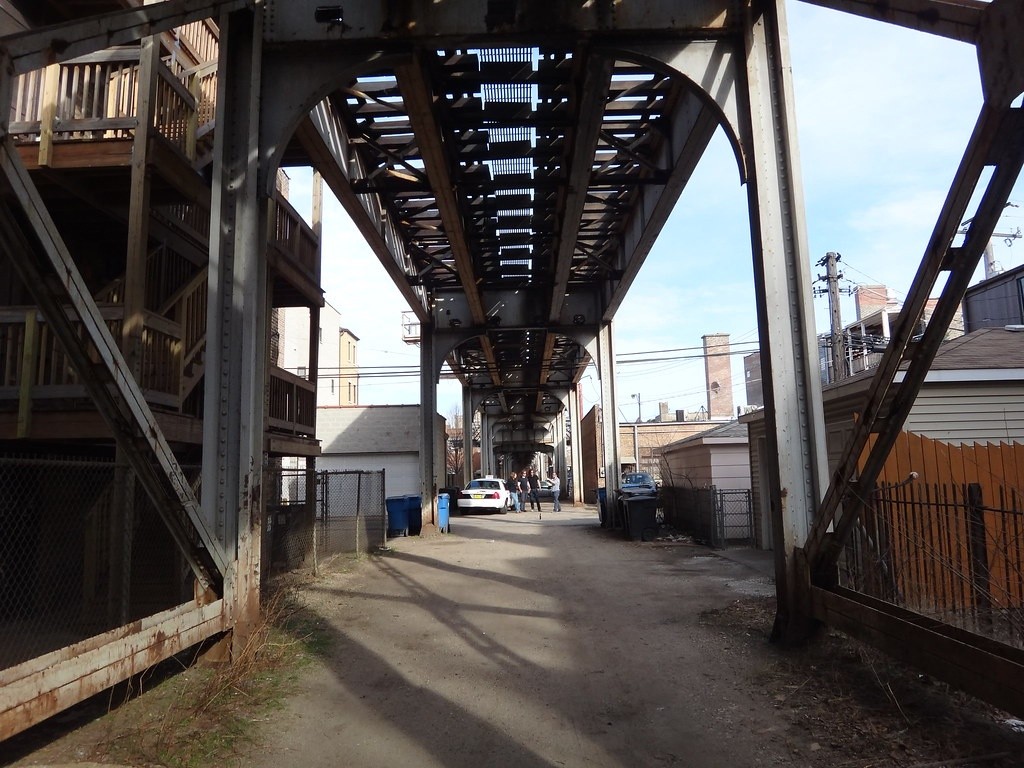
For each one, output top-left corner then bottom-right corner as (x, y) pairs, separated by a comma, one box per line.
(624, 496), (658, 540)
(439, 487), (460, 516)
(595, 487), (607, 528)
(438, 493), (450, 533)
(614, 489), (658, 532)
(385, 494), (422, 536)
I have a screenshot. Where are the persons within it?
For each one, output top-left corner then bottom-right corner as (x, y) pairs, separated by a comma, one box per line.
(527, 469), (543, 512)
(545, 472), (561, 512)
(518, 470), (531, 512)
(506, 472), (521, 513)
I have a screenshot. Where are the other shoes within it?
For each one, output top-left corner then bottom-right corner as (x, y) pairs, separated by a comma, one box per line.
(522, 509), (526, 512)
(530, 509), (533, 512)
(538, 510), (542, 512)
(516, 509), (521, 513)
(553, 509), (561, 512)
(520, 507), (523, 511)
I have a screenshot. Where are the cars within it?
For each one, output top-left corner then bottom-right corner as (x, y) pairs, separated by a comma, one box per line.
(621, 472), (656, 492)
(457, 474), (516, 514)
